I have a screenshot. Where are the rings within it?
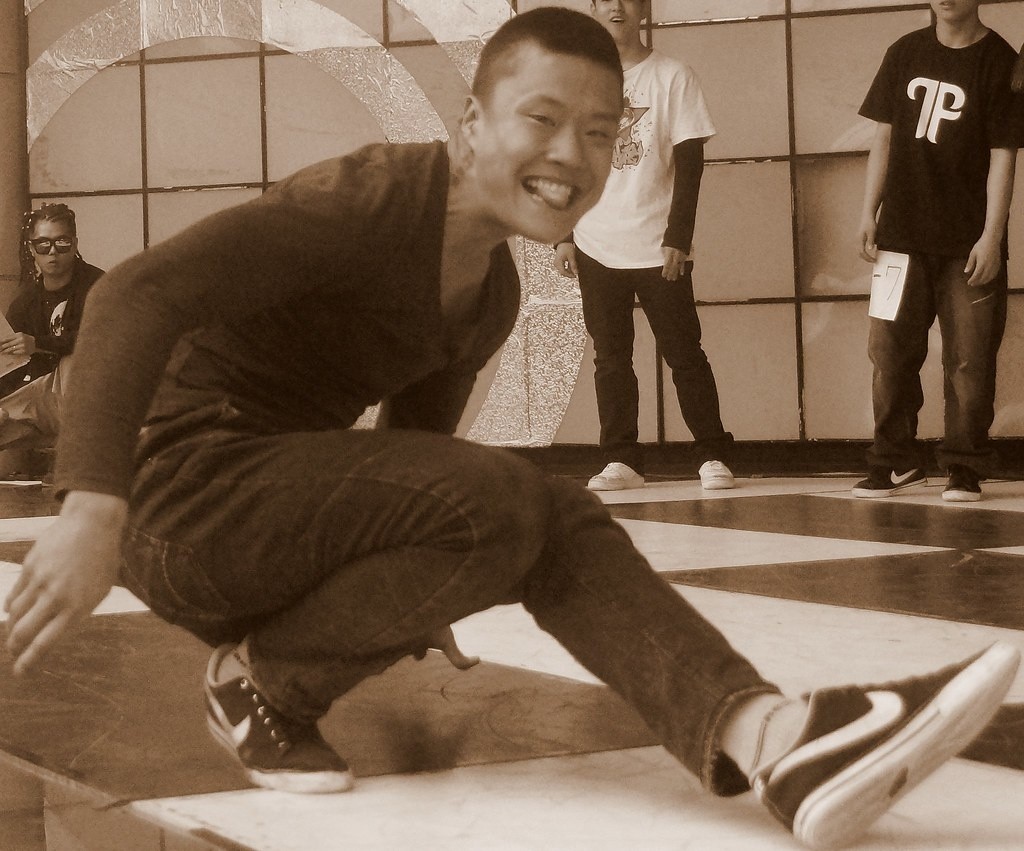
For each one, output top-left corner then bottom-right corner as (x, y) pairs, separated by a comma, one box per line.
(14, 346), (15, 350)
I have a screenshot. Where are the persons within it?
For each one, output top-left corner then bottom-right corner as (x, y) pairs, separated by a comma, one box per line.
(3, 7), (1019, 851)
(852, 1), (1023, 503)
(552, 1), (740, 491)
(0, 204), (106, 487)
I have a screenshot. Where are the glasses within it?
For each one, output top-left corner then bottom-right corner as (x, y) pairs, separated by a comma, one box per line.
(27, 236), (72, 254)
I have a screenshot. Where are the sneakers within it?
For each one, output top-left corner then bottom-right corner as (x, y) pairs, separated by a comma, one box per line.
(588, 462), (644, 490)
(853, 464), (929, 498)
(747, 639), (1022, 848)
(942, 463), (982, 502)
(699, 460), (735, 489)
(205, 642), (353, 793)
